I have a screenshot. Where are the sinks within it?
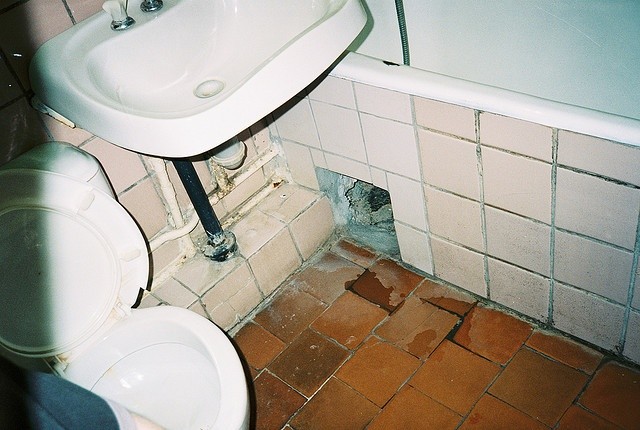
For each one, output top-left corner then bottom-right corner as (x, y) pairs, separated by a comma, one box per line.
(27, 0), (370, 161)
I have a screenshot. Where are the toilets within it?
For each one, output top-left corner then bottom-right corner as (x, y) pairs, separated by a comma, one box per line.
(0, 141), (252, 429)
